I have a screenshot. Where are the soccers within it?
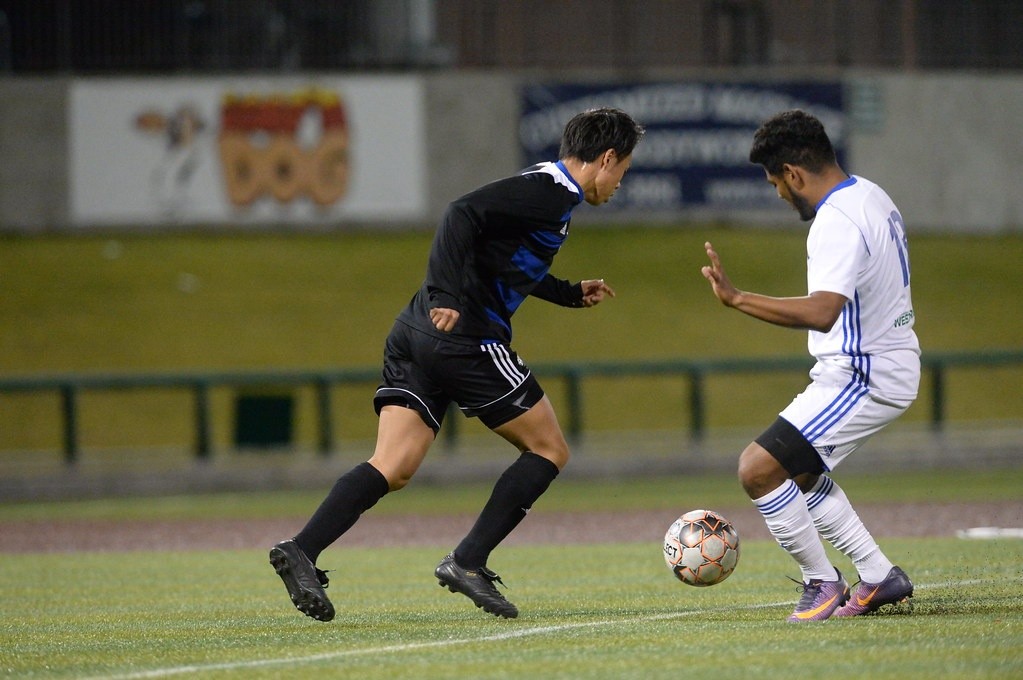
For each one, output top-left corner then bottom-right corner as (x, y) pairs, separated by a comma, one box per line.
(663, 509), (740, 587)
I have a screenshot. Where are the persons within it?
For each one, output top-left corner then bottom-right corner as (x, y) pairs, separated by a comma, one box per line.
(702, 110), (922, 619)
(270, 108), (640, 622)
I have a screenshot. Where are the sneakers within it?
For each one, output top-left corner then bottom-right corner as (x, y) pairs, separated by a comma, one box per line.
(435, 549), (518, 619)
(832, 565), (914, 618)
(269, 539), (335, 622)
(786, 565), (850, 623)
